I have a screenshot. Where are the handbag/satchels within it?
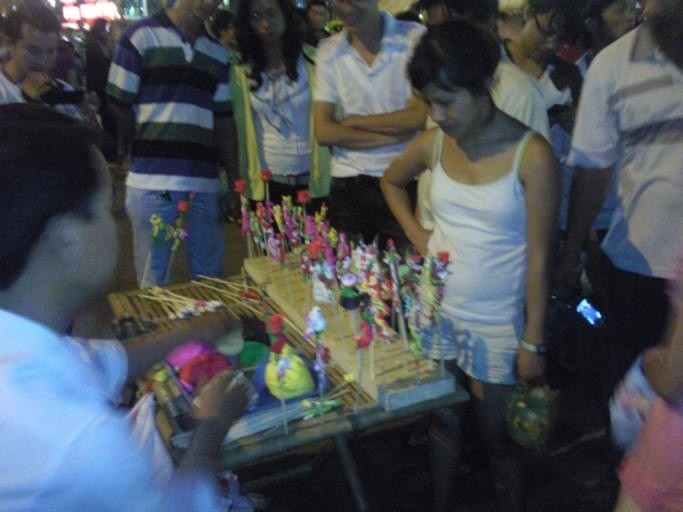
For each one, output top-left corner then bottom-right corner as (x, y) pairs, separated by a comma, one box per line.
(507, 380), (560, 463)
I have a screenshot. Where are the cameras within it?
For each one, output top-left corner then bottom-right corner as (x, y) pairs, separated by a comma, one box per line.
(40, 78), (82, 105)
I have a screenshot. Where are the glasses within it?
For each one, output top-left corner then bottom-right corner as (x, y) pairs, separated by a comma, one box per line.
(531, 12), (564, 53)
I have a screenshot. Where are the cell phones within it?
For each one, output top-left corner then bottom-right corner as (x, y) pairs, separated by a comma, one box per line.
(547, 104), (564, 117)
(575, 297), (602, 325)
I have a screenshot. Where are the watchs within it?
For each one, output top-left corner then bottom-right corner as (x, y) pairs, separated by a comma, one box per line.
(520, 339), (547, 354)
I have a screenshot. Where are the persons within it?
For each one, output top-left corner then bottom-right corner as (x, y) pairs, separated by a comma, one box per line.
(583, 0), (636, 72)
(380, 21), (564, 512)
(305, 0), (332, 48)
(213, 11), (247, 222)
(604, 287), (683, 512)
(65, 15), (110, 100)
(105, 0), (234, 285)
(313, 0), (432, 242)
(228, 0), (330, 210)
(554, 0), (683, 368)
(417, 0), (553, 232)
(0, 0), (91, 127)
(0, 103), (250, 512)
(500, 0), (591, 257)
